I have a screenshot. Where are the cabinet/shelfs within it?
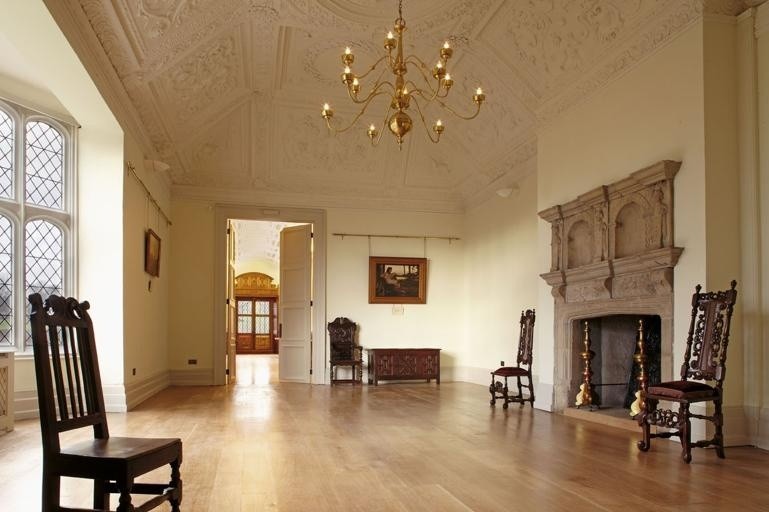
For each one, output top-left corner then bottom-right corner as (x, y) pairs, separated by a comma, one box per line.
(364, 348), (443, 386)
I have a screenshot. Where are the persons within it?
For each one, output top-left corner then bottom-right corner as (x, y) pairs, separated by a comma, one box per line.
(380, 267), (416, 292)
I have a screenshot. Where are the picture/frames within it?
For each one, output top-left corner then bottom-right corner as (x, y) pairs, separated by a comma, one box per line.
(368, 255), (428, 304)
(145, 228), (162, 277)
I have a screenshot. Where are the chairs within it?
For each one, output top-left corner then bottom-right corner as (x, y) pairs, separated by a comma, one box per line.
(636, 280), (739, 462)
(27, 292), (183, 512)
(489, 307), (536, 410)
(327, 317), (363, 387)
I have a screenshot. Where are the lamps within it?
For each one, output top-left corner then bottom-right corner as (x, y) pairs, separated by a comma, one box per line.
(321, 1), (487, 155)
